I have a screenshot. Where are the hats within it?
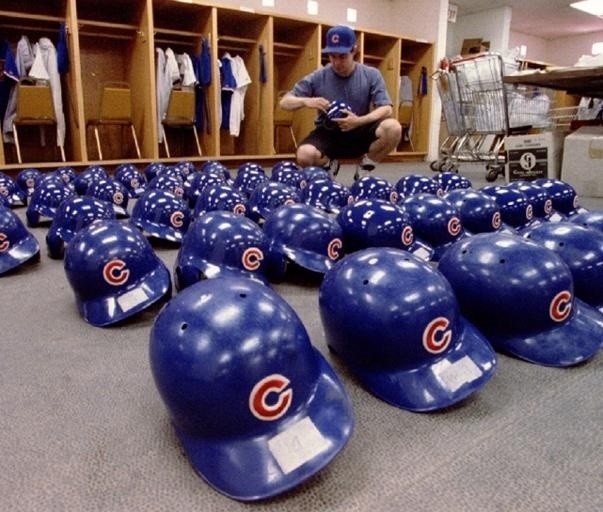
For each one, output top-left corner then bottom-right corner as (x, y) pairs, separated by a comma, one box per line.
(324, 97), (362, 131)
(322, 26), (356, 54)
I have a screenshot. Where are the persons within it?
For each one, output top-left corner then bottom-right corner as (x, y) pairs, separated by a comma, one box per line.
(280, 26), (402, 181)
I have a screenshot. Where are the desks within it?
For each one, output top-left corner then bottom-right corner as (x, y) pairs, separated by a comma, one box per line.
(505, 65), (603, 89)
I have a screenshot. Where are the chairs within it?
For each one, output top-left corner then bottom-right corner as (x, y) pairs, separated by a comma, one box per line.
(12, 78), (67, 164)
(398, 100), (415, 151)
(86, 82), (142, 160)
(161, 90), (203, 157)
(274, 90), (299, 153)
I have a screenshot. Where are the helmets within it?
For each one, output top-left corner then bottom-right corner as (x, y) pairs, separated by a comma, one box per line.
(337, 198), (433, 263)
(65, 218), (170, 326)
(261, 204), (343, 276)
(45, 196), (116, 259)
(445, 189), (517, 237)
(173, 211), (274, 292)
(437, 209), (603, 368)
(402, 192), (472, 246)
(484, 183), (543, 234)
(0, 211), (40, 274)
(1, 160), (582, 220)
(129, 189), (190, 244)
(148, 276), (355, 502)
(319, 246), (498, 413)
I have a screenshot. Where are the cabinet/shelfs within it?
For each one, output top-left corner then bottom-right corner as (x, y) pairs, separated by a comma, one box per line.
(0, 0), (435, 170)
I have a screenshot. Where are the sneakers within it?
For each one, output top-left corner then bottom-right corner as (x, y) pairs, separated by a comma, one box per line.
(354, 163), (376, 180)
(322, 159), (341, 177)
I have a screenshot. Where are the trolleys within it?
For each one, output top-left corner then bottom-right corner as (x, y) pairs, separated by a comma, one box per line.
(430, 52), (587, 182)
